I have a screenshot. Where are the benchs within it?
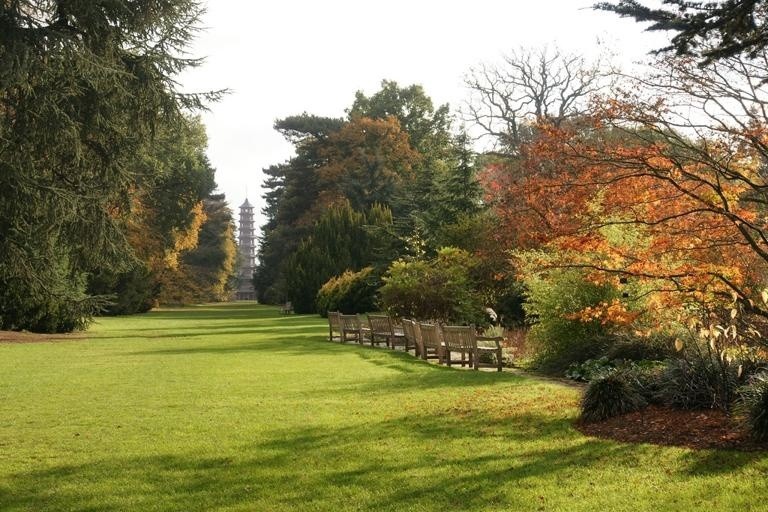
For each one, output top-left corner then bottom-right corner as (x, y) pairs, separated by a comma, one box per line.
(327, 310), (505, 372)
